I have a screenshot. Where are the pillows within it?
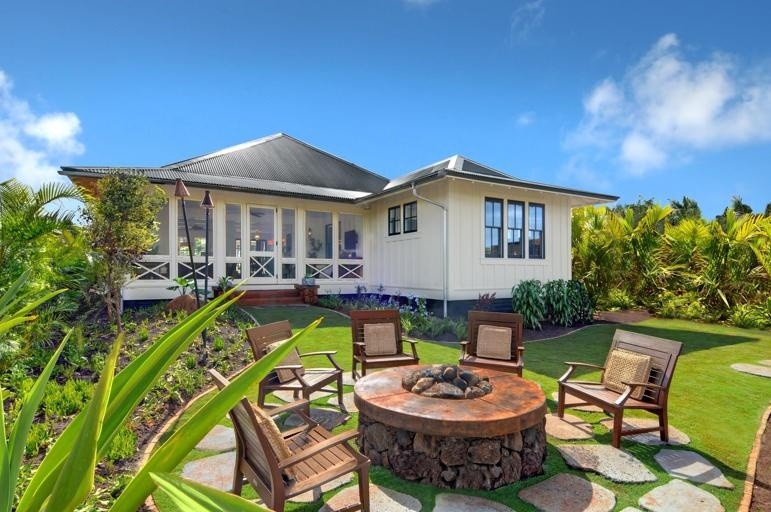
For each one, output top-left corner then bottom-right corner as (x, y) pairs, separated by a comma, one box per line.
(475, 325), (512, 361)
(602, 347), (654, 401)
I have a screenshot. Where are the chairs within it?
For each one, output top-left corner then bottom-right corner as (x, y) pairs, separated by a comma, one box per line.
(350, 308), (419, 379)
(246, 319), (344, 419)
(210, 368), (373, 511)
(557, 328), (684, 451)
(459, 309), (526, 379)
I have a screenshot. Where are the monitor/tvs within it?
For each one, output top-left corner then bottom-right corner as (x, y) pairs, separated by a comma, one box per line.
(344, 230), (356, 249)
